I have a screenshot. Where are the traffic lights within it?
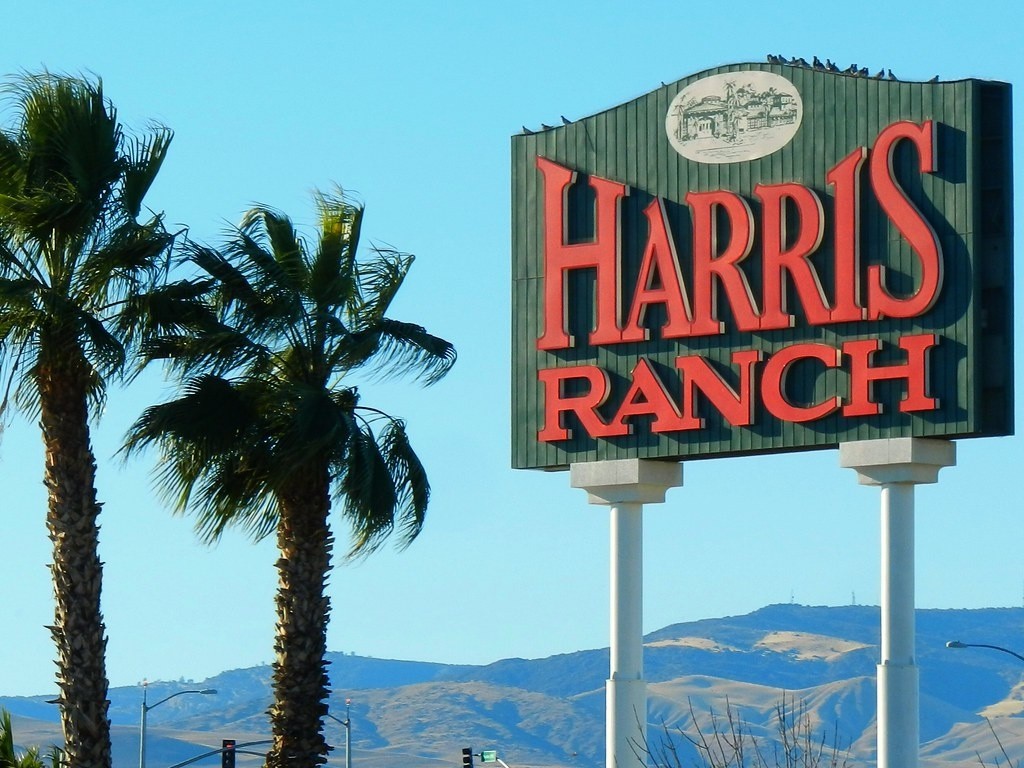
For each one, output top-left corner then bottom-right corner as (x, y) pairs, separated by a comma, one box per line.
(222, 740), (236, 768)
(462, 747), (473, 768)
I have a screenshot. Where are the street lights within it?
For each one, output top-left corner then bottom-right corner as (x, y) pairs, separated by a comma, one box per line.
(141, 682), (217, 768)
(946, 640), (1023, 662)
